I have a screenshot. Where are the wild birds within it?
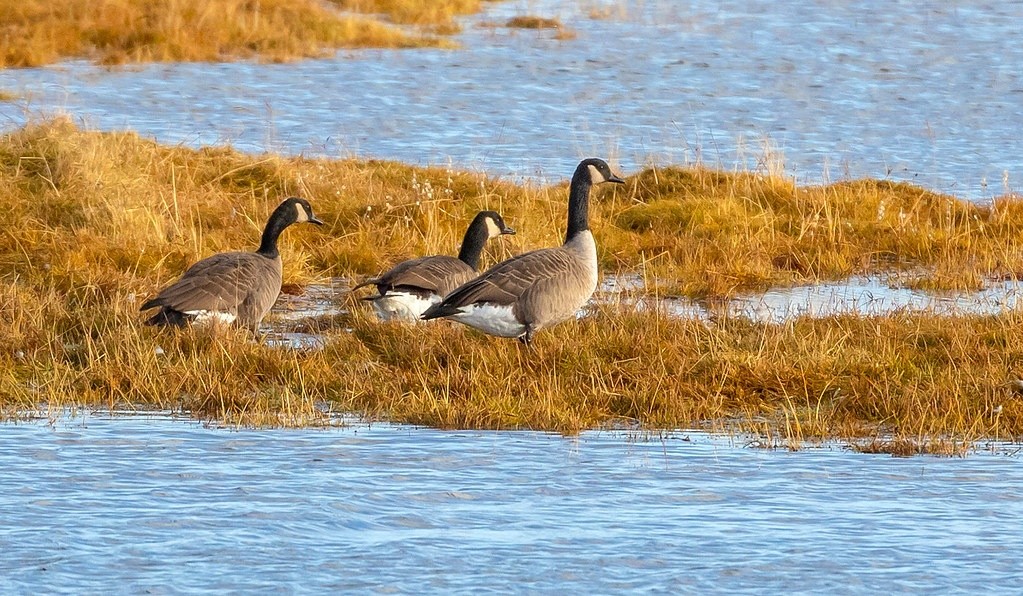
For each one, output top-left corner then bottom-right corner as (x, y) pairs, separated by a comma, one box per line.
(355, 158), (627, 345)
(141, 197), (325, 344)
